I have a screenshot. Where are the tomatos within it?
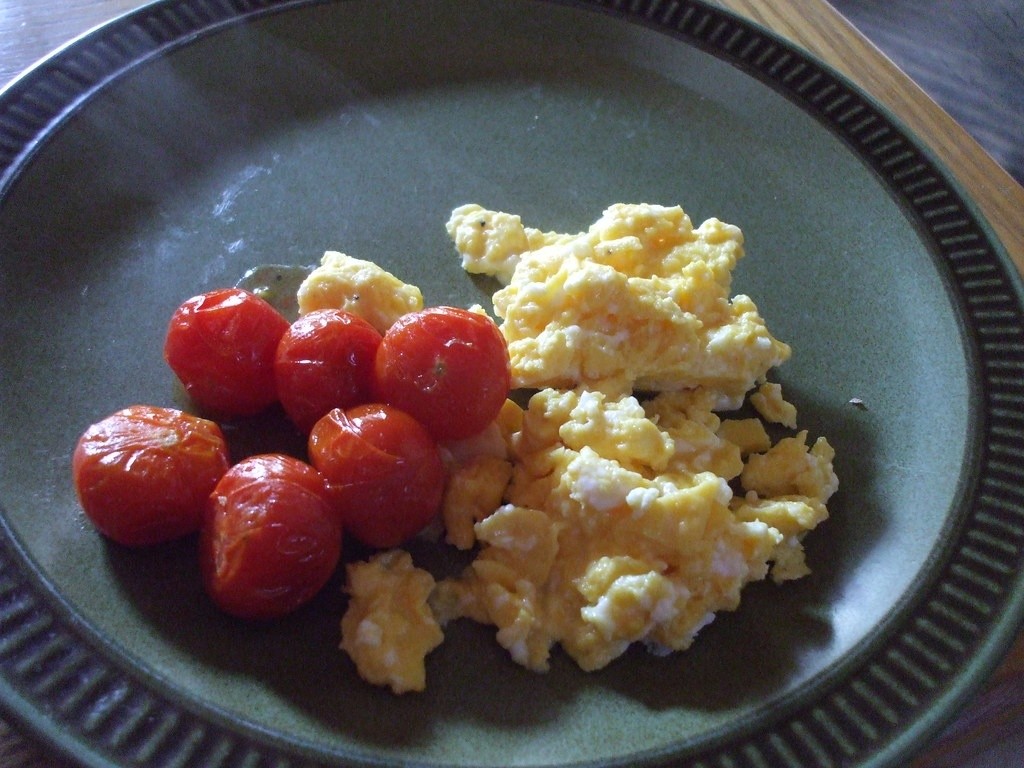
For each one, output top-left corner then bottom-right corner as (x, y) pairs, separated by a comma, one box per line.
(73, 287), (510, 616)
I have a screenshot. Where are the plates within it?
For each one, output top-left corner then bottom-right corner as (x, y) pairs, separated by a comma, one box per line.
(0, 0), (1024, 768)
